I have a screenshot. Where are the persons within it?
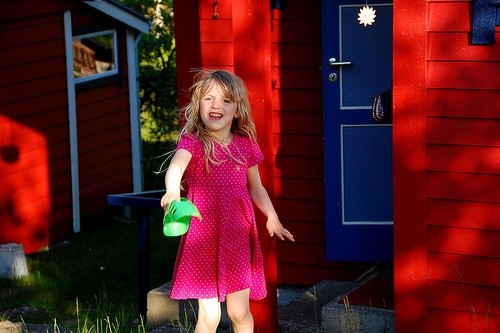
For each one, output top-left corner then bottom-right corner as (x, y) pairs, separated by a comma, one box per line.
(161, 70), (296, 333)
